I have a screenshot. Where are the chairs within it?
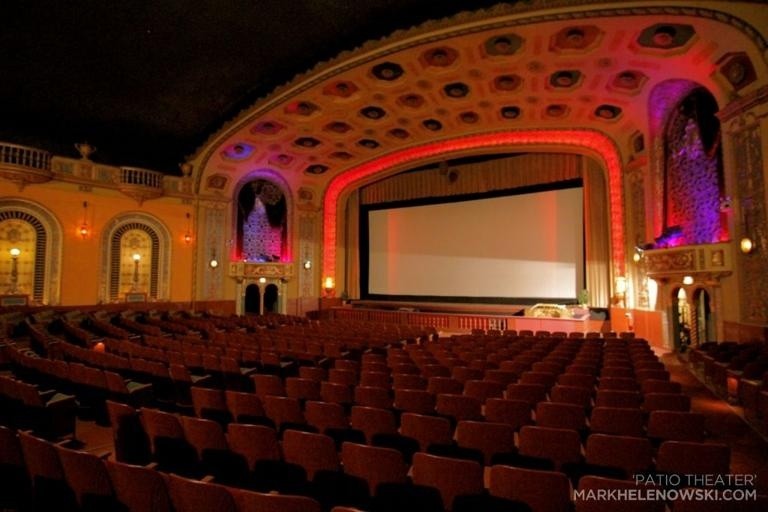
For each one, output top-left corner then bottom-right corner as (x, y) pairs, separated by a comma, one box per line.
(0, 291), (768, 510)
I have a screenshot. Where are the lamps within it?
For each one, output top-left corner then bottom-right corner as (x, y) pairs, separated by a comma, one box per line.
(738, 236), (759, 255)
